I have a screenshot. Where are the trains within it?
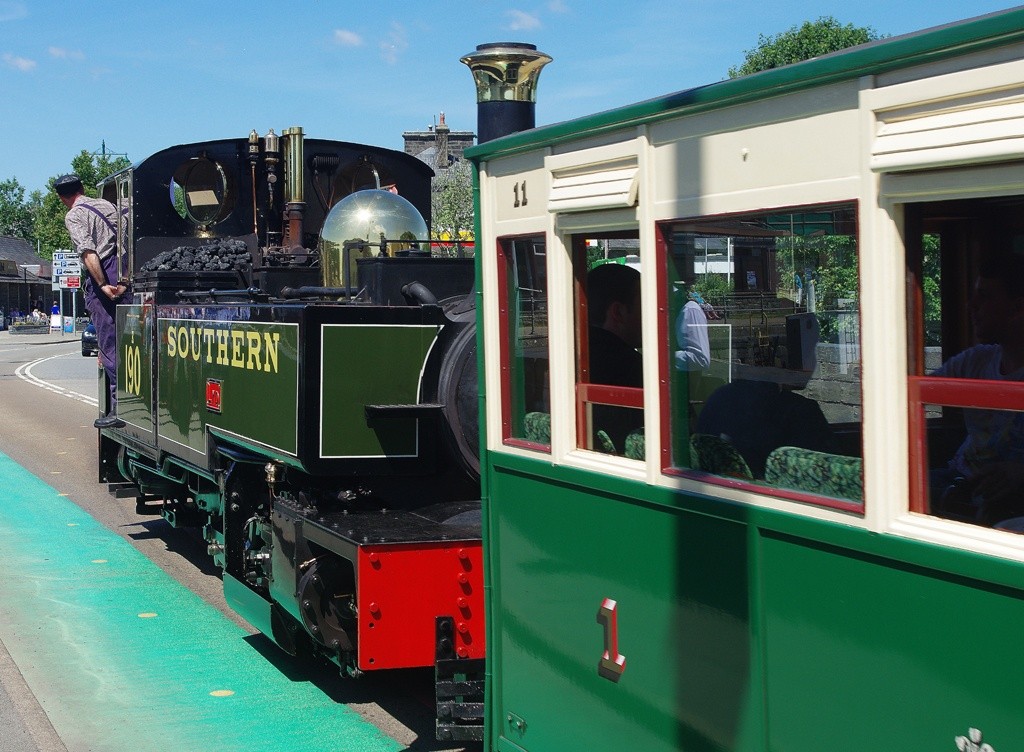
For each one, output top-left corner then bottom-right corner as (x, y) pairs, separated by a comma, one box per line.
(90, 5), (1023, 752)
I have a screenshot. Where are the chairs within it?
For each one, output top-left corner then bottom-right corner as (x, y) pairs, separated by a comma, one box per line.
(766, 446), (863, 502)
(682, 431), (751, 481)
(524, 411), (645, 460)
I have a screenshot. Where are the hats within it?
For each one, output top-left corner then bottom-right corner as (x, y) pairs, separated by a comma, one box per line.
(54, 175), (83, 197)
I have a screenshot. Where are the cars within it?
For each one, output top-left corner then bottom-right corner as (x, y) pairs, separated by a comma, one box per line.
(81, 317), (98, 357)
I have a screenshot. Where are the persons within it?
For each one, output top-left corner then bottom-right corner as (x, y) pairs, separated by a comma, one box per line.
(52, 175), (128, 428)
(534, 264), (641, 453)
(697, 298), (834, 482)
(51, 301), (60, 328)
(667, 259), (721, 468)
(790, 272), (802, 303)
(925, 248), (1024, 526)
(0, 306), (36, 322)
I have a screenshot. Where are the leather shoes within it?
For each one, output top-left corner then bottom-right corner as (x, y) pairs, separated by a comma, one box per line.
(93, 411), (120, 429)
